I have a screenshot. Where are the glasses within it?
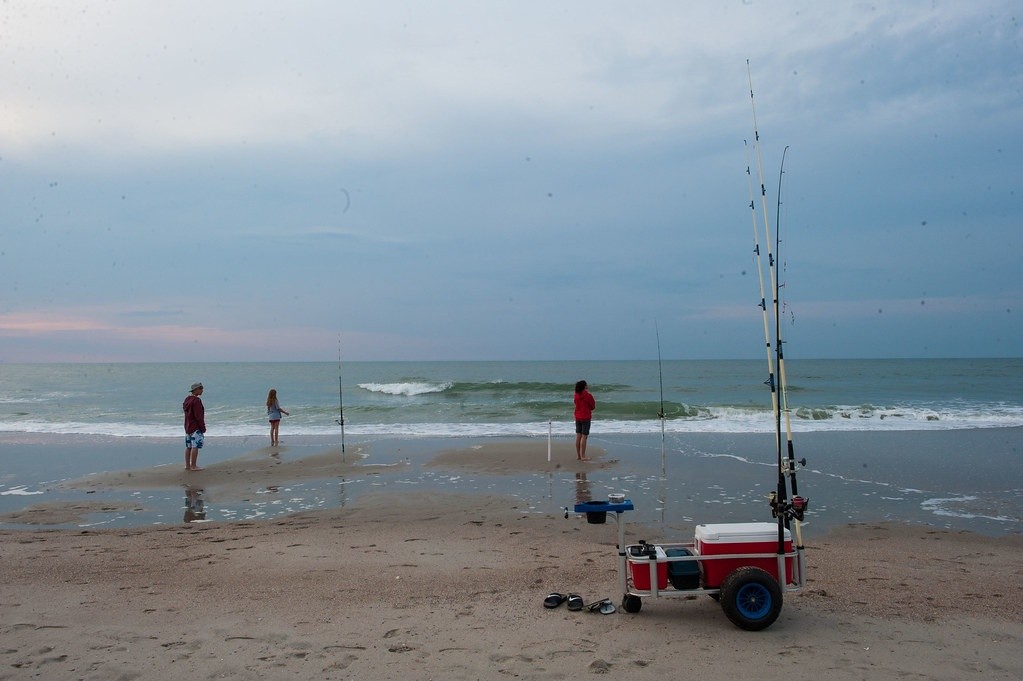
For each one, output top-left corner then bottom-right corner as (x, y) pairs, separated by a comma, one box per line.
(196, 387), (203, 390)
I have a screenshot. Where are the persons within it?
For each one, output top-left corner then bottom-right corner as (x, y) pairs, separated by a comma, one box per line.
(181, 383), (208, 471)
(182, 490), (206, 522)
(572, 380), (597, 461)
(265, 388), (289, 446)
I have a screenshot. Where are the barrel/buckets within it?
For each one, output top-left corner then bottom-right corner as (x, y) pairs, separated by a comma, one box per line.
(585, 501), (608, 524)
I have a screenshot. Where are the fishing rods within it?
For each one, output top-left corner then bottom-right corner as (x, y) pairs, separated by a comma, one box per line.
(744, 58), (810, 555)
(337, 330), (345, 464)
(654, 318), (666, 477)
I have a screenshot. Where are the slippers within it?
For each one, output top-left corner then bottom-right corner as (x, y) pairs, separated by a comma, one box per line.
(568, 593), (583, 611)
(586, 597), (615, 614)
(543, 592), (567, 609)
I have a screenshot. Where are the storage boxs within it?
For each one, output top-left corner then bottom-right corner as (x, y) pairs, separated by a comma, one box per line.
(694, 522), (794, 587)
(628, 545), (667, 591)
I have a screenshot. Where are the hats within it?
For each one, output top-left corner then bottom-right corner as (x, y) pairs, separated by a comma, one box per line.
(188, 382), (203, 392)
